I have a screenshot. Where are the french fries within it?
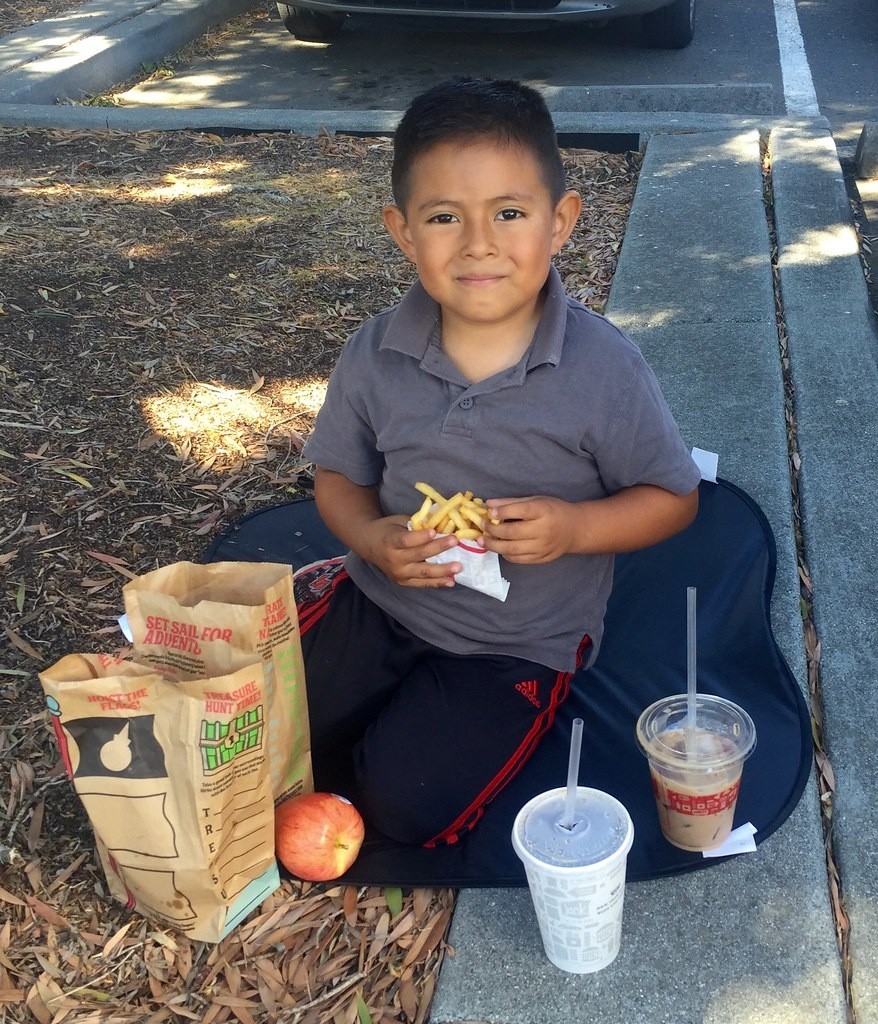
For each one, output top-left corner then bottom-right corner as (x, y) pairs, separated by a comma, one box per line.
(407, 482), (500, 548)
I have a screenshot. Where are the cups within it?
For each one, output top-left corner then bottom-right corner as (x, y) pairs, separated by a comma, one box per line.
(512, 786), (635, 973)
(636, 694), (758, 850)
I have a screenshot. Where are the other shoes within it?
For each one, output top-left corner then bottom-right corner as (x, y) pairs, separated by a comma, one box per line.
(293, 555), (348, 602)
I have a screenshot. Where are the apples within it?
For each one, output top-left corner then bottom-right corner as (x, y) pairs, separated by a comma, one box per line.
(272, 792), (364, 882)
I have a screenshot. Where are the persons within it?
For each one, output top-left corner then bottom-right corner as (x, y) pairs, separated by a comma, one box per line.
(293, 76), (702, 849)
